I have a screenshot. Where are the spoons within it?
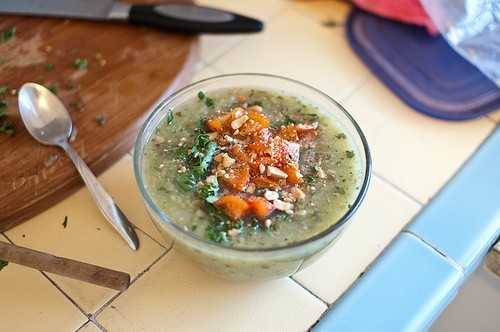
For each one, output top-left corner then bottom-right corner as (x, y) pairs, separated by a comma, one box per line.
(17, 83), (141, 252)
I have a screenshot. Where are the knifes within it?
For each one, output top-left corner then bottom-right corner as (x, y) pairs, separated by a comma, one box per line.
(1, 1), (265, 34)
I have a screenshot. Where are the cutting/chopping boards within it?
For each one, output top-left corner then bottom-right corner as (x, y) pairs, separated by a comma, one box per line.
(0, 1), (210, 238)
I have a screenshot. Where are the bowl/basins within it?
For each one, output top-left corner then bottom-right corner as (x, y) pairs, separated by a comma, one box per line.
(133, 71), (371, 285)
